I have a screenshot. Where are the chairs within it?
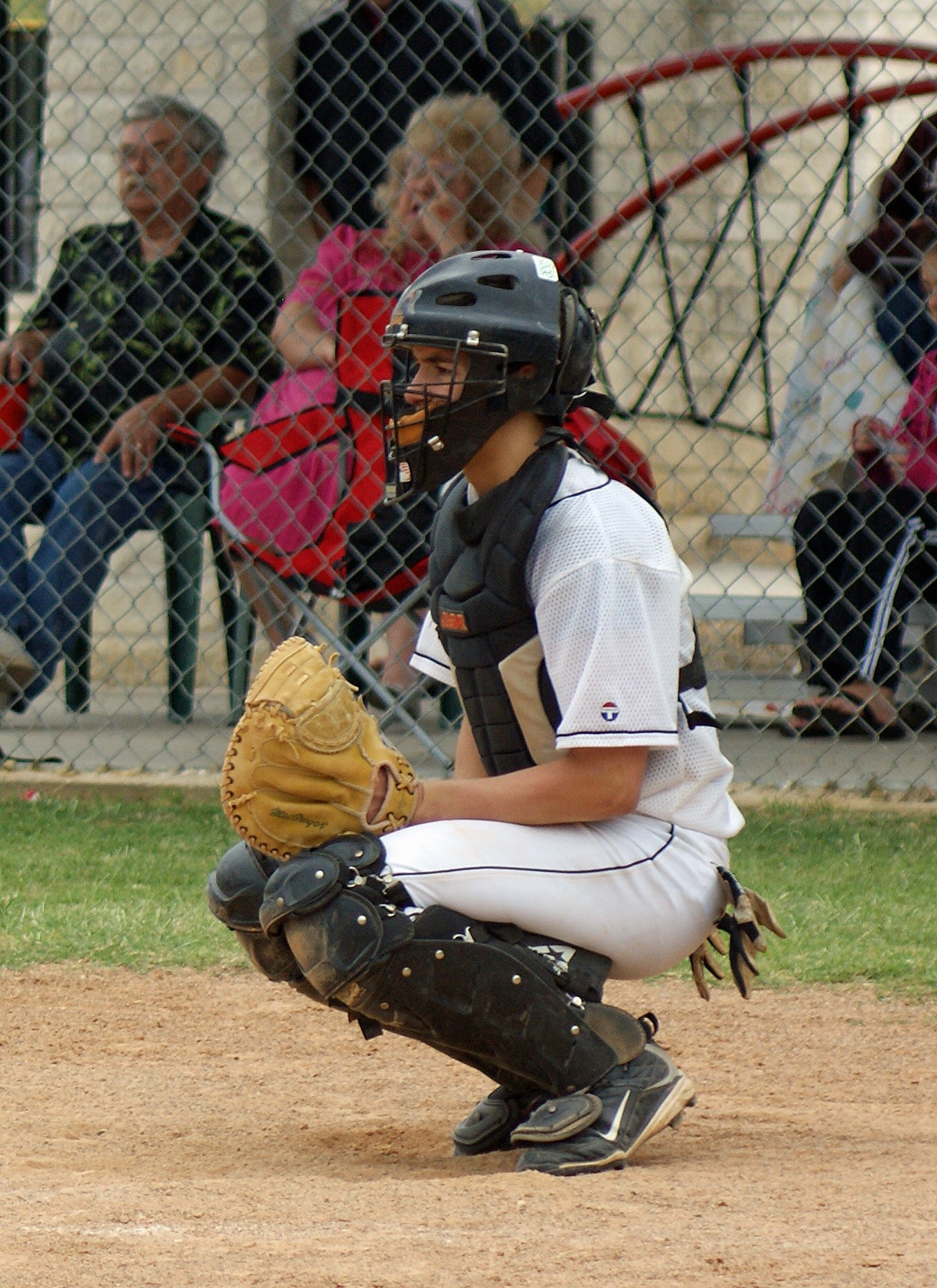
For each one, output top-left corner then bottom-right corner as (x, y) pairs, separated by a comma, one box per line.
(64, 406), (259, 726)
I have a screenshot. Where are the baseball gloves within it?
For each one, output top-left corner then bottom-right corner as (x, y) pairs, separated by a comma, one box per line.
(222, 636), (419, 862)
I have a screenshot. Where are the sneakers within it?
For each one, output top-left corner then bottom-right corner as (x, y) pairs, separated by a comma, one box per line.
(453, 1084), (546, 1156)
(514, 1041), (698, 1176)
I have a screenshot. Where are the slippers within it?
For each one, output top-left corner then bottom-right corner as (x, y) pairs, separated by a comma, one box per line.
(780, 720), (850, 739)
(791, 689), (906, 741)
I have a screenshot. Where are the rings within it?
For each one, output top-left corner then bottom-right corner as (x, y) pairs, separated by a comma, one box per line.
(137, 443), (144, 448)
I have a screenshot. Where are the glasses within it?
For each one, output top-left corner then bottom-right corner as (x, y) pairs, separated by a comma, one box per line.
(113, 145), (203, 166)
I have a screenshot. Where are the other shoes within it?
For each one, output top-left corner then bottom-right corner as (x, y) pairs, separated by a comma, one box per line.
(370, 684), (423, 720)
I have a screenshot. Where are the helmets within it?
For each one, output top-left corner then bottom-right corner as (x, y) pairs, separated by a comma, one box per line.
(379, 250), (599, 506)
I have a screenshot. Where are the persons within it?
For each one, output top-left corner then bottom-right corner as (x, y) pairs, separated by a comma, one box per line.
(0, 97), (286, 715)
(768, 112), (937, 738)
(286, 0), (569, 234)
(202, 250), (745, 1173)
(277, 92), (656, 736)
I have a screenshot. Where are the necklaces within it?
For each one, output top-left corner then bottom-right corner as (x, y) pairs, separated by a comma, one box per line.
(443, 244), (471, 260)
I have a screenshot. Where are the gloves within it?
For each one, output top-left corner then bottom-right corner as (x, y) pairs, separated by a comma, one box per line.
(715, 865), (786, 1001)
(689, 929), (727, 1002)
